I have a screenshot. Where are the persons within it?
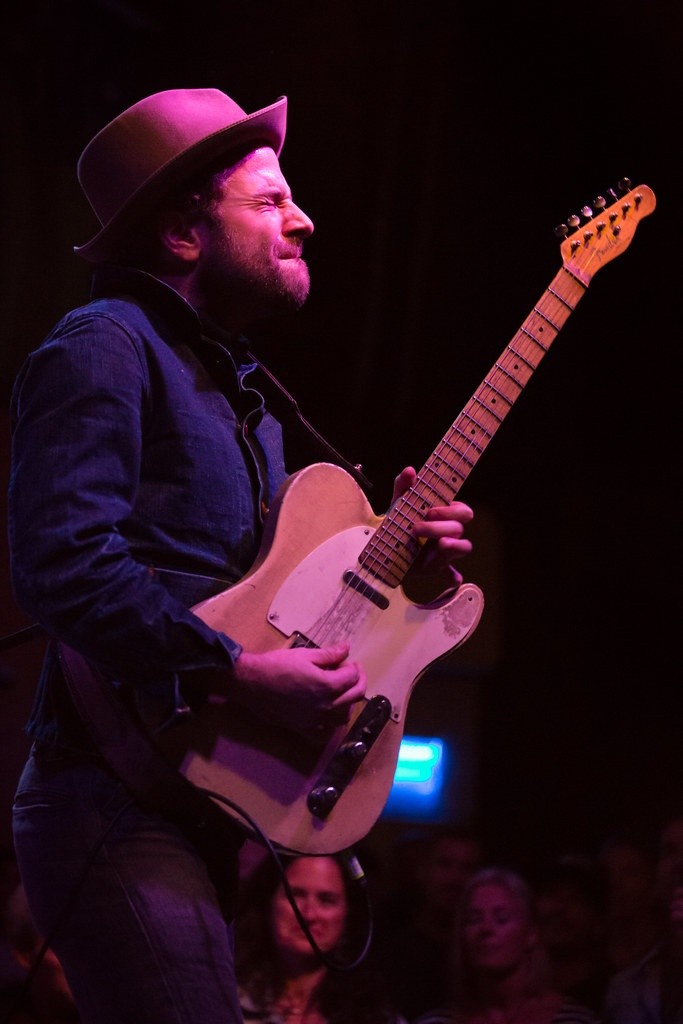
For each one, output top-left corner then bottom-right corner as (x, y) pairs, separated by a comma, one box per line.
(0, 92), (474, 1024)
(0, 821), (683, 1024)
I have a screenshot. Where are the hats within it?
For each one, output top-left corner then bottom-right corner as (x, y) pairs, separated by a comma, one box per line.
(73, 88), (287, 263)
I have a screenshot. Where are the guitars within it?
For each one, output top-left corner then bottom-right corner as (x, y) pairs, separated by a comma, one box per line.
(172, 176), (657, 859)
